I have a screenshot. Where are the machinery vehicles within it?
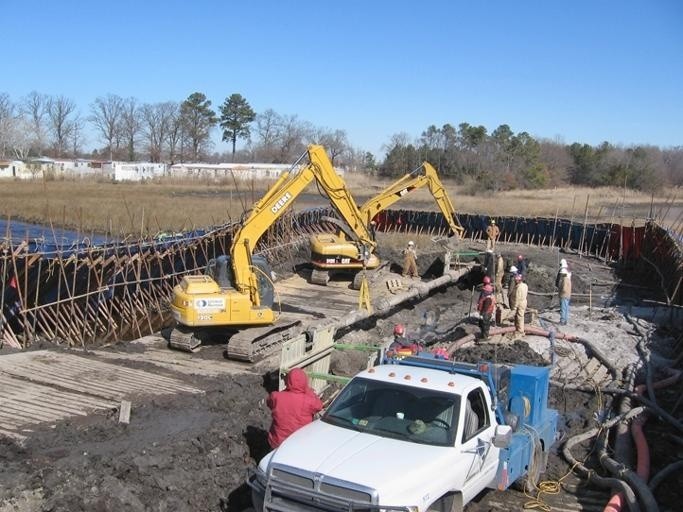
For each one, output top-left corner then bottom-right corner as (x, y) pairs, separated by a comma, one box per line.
(158, 140), (382, 366)
(307, 154), (469, 290)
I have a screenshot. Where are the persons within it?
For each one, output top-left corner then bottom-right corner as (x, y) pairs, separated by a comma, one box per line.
(485, 220), (500, 254)
(387, 321), (424, 360)
(264, 369), (323, 452)
(473, 252), (575, 343)
(401, 240), (419, 277)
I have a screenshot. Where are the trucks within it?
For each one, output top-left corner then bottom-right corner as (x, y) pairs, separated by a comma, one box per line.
(245, 350), (569, 512)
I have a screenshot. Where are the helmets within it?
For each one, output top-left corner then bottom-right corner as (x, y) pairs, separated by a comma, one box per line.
(517, 254), (524, 262)
(490, 220), (496, 224)
(481, 285), (494, 293)
(482, 276), (491, 283)
(393, 324), (405, 334)
(559, 258), (569, 275)
(407, 240), (415, 246)
(508, 265), (519, 273)
(514, 274), (523, 281)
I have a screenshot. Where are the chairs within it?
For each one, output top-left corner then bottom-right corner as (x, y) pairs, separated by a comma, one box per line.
(419, 396), (479, 440)
(371, 390), (419, 419)
